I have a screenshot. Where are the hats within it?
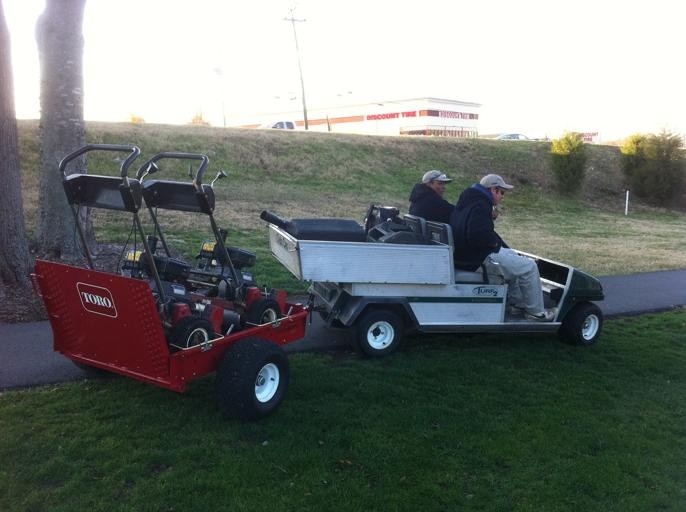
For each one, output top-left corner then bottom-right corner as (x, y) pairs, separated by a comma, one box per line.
(481, 173), (514, 191)
(421, 170), (452, 185)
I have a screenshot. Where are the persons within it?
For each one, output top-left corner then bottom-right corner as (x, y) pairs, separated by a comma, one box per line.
(450, 173), (555, 321)
(409, 170), (498, 224)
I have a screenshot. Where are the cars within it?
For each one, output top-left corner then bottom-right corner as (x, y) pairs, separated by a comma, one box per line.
(257, 119), (296, 131)
(493, 132), (529, 141)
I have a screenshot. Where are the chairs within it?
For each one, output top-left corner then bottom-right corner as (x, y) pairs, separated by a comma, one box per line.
(402, 211), (426, 242)
(423, 219), (505, 285)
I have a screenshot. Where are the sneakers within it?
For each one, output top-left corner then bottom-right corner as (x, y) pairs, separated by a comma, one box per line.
(511, 304), (525, 315)
(526, 306), (559, 323)
